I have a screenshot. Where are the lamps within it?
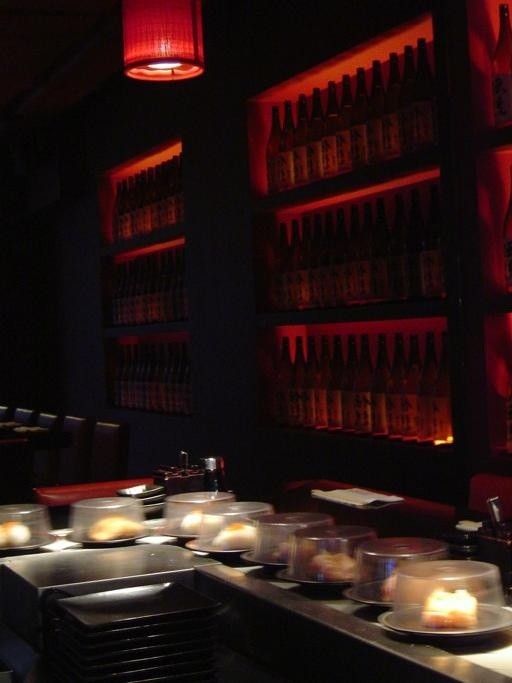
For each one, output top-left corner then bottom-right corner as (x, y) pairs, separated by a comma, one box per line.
(120, 0), (207, 83)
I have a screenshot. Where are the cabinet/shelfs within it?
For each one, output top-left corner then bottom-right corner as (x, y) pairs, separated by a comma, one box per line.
(93, 0), (512, 460)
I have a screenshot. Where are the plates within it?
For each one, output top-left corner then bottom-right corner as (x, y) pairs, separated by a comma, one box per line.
(0, 522), (511, 636)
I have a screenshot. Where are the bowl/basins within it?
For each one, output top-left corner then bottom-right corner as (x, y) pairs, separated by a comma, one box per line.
(117, 483), (167, 515)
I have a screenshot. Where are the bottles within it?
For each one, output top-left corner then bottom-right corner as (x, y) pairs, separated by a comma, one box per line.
(490, 3), (512, 128)
(204, 456), (234, 493)
(463, 496), (512, 605)
(263, 31), (442, 444)
(111, 154), (194, 427)
